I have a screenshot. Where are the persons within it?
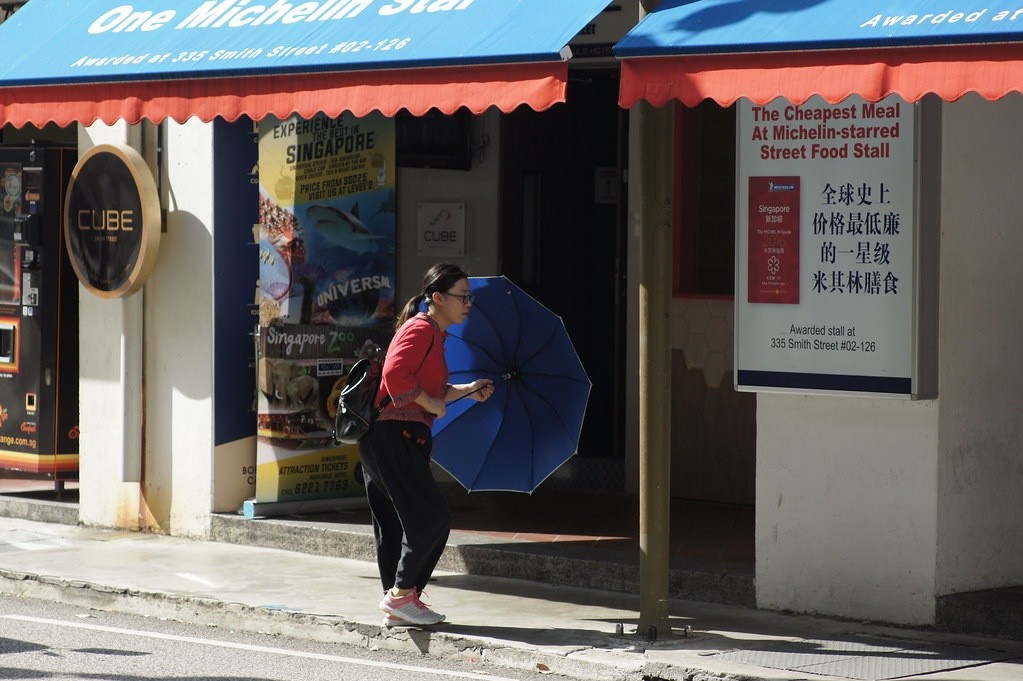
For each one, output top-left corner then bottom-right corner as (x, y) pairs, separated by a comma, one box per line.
(358, 263), (494, 627)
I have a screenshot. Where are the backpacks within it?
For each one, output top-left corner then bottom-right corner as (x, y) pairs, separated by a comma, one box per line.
(334, 318), (434, 444)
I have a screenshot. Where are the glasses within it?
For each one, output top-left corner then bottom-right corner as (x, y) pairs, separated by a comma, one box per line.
(431, 290), (475, 305)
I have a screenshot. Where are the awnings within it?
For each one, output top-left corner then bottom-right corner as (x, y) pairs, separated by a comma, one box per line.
(0, 0), (612, 128)
(612, 0), (1023, 110)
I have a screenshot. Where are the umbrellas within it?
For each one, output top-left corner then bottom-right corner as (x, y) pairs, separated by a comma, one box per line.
(415, 274), (593, 496)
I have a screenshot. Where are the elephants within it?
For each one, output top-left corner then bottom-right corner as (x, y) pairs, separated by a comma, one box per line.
(272, 360), (320, 410)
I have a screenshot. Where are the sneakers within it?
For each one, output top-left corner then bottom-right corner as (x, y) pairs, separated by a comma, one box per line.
(378, 586), (439, 625)
(383, 603), (446, 627)
